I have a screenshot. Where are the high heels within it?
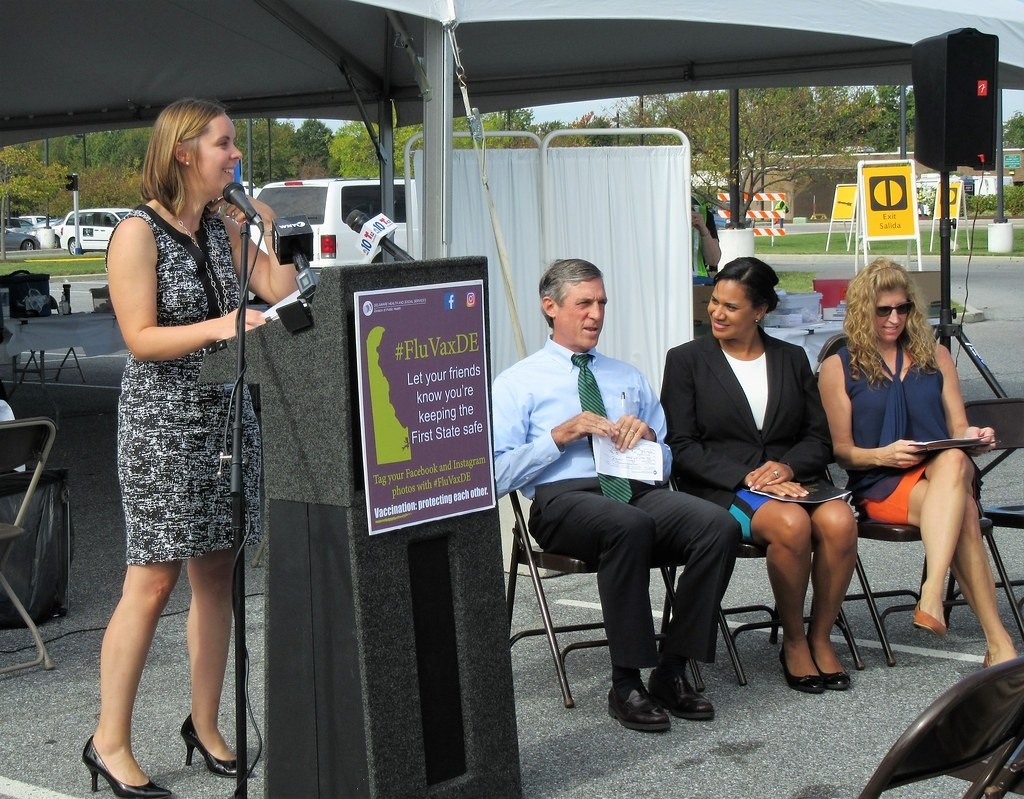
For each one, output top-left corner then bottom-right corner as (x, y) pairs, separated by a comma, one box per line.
(82, 736), (172, 799)
(180, 714), (249, 778)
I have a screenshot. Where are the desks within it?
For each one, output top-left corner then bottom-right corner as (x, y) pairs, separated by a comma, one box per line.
(0, 310), (127, 402)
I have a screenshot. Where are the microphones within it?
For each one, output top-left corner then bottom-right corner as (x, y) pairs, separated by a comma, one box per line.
(223, 182), (264, 233)
(270, 214), (319, 304)
(346, 210), (416, 262)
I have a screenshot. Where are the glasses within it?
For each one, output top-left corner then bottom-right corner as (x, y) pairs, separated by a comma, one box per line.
(877, 297), (914, 316)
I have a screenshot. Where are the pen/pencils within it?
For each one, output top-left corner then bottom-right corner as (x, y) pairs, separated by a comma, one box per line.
(621, 392), (625, 399)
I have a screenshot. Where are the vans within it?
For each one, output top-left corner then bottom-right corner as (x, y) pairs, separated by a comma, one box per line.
(60, 207), (132, 256)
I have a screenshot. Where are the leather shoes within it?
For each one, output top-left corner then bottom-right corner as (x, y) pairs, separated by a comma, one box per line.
(607, 683), (671, 731)
(647, 670), (714, 720)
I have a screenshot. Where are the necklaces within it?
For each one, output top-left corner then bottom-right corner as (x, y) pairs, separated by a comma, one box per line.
(173, 214), (229, 317)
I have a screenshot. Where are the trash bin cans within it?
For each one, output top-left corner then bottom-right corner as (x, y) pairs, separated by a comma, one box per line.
(1, 468), (74, 629)
(9, 274), (50, 317)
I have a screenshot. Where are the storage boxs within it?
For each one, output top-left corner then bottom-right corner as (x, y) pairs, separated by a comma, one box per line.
(906, 269), (941, 319)
(812, 278), (853, 309)
(89, 287), (114, 313)
(693, 285), (715, 326)
(0, 270), (51, 318)
(775, 291), (823, 323)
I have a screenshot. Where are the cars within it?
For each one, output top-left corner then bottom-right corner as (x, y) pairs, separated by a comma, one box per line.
(22, 216), (63, 229)
(712, 201), (751, 229)
(2, 228), (40, 251)
(5, 218), (34, 233)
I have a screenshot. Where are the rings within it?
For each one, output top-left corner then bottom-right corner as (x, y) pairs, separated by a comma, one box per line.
(231, 211), (237, 216)
(773, 470), (780, 478)
(629, 430), (635, 435)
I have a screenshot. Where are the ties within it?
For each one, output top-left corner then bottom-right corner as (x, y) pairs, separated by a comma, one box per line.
(571, 353), (633, 504)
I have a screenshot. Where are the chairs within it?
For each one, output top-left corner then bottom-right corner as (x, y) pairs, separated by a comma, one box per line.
(0, 417), (59, 675)
(813, 333), (1024, 668)
(667, 465), (865, 686)
(943, 397), (1024, 632)
(506, 487), (704, 710)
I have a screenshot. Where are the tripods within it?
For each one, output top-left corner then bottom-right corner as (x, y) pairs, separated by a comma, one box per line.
(932, 170), (1016, 478)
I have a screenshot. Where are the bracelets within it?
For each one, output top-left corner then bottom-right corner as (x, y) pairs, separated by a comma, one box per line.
(264, 228), (273, 236)
(700, 228), (709, 236)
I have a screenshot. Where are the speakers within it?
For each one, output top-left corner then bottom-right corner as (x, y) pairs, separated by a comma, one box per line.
(911, 28), (1000, 174)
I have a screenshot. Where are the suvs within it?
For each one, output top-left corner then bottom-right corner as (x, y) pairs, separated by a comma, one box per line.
(256, 178), (409, 266)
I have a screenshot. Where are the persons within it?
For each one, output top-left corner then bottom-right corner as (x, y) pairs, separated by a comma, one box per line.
(819, 259), (1016, 670)
(691, 196), (721, 284)
(80, 98), (300, 799)
(660, 258), (856, 693)
(491, 259), (742, 730)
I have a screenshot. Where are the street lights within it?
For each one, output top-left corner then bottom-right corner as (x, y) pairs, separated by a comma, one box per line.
(73, 135), (86, 169)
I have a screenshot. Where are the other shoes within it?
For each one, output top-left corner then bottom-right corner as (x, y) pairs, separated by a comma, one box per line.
(982, 649), (991, 668)
(778, 645), (825, 693)
(912, 599), (946, 638)
(807, 639), (849, 690)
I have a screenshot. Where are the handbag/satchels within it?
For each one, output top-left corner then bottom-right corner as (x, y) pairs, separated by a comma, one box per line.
(0, 270), (52, 319)
